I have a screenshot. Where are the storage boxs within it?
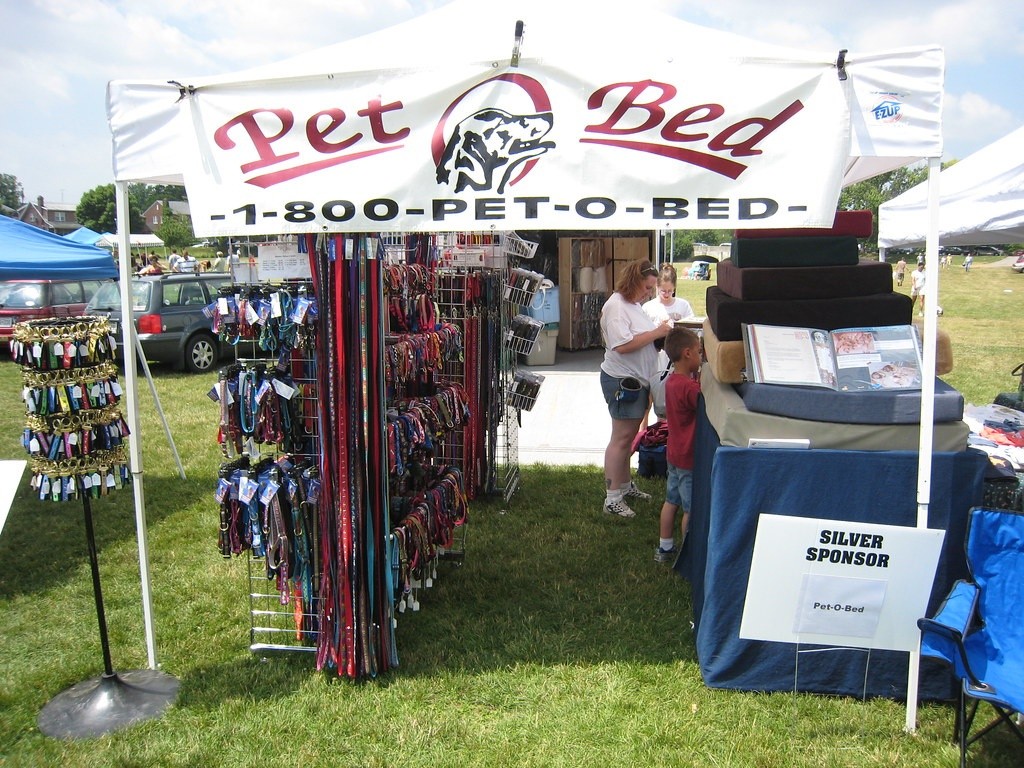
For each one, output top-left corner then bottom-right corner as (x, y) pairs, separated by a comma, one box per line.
(638, 446), (668, 477)
(519, 285), (561, 365)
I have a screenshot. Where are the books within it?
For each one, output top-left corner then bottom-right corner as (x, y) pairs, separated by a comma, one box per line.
(739, 321), (923, 392)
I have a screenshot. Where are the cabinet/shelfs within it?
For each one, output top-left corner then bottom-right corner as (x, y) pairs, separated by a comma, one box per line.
(559, 237), (614, 349)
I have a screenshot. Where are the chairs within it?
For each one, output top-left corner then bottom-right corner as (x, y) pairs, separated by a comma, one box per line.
(177, 286), (203, 308)
(915, 505), (1024, 768)
(78, 289), (94, 303)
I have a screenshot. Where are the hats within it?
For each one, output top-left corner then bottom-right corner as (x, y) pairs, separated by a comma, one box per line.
(984, 456), (1019, 481)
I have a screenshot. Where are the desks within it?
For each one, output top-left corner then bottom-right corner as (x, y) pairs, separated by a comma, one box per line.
(683, 387), (987, 701)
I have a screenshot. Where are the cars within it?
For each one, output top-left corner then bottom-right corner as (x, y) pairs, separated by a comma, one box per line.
(0, 279), (122, 349)
(1012, 254), (1024, 273)
(81, 271), (236, 376)
(887, 247), (1004, 256)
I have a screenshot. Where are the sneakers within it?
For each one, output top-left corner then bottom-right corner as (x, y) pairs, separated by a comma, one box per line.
(653, 544), (679, 562)
(602, 496), (636, 518)
(622, 481), (652, 499)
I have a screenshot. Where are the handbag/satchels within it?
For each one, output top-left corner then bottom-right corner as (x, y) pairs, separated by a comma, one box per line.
(1011, 363), (1024, 401)
(637, 443), (667, 477)
(648, 369), (671, 419)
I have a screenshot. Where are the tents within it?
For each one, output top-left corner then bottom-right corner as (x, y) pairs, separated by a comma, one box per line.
(0, 214), (189, 481)
(64, 226), (102, 246)
(875, 126), (1022, 265)
(101, 0), (946, 730)
(95, 232), (165, 268)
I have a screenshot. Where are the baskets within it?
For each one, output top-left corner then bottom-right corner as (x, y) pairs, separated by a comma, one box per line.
(503, 268), (544, 307)
(504, 370), (545, 412)
(504, 314), (544, 355)
(503, 235), (540, 258)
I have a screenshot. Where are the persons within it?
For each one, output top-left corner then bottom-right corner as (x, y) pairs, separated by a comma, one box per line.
(917, 253), (923, 265)
(896, 257), (911, 286)
(652, 328), (704, 562)
(964, 253), (973, 271)
(227, 249), (240, 272)
(639, 262), (695, 431)
(131, 251), (168, 274)
(249, 254), (256, 266)
(911, 264), (926, 315)
(941, 252), (952, 268)
(168, 248), (181, 271)
(173, 250), (201, 274)
(598, 258), (678, 519)
(213, 251), (226, 273)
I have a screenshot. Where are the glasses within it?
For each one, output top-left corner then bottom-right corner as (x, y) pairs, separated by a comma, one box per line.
(658, 287), (674, 294)
